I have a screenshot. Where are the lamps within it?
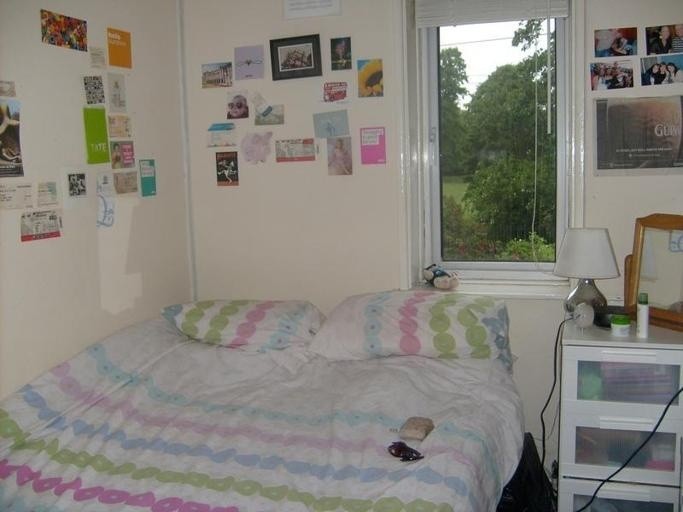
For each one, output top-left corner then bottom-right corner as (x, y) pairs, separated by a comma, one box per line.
(553, 226), (621, 318)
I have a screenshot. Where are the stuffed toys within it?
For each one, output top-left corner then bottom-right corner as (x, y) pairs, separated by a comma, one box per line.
(424, 262), (460, 289)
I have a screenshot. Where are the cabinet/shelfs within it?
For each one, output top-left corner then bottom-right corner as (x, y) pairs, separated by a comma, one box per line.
(555, 315), (682, 510)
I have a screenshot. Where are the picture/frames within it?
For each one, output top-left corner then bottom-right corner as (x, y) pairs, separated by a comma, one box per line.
(269, 33), (323, 81)
(586, 22), (683, 177)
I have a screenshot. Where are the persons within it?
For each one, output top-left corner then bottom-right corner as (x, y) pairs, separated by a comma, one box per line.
(227, 94), (249, 118)
(593, 61), (632, 90)
(649, 25), (683, 55)
(329, 139), (351, 174)
(643, 61), (683, 85)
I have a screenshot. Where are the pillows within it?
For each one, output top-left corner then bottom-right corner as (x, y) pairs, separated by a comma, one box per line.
(307, 292), (509, 366)
(162, 301), (327, 349)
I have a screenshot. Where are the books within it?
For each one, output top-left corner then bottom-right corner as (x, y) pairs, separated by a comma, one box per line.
(601, 361), (674, 403)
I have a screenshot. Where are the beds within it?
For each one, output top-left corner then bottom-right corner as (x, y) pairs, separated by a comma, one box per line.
(0, 309), (517, 512)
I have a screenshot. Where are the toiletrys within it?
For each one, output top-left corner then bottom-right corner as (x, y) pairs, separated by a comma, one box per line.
(636, 293), (649, 339)
(611, 314), (631, 337)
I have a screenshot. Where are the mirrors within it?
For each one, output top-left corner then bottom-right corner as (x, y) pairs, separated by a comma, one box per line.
(624, 212), (683, 331)
